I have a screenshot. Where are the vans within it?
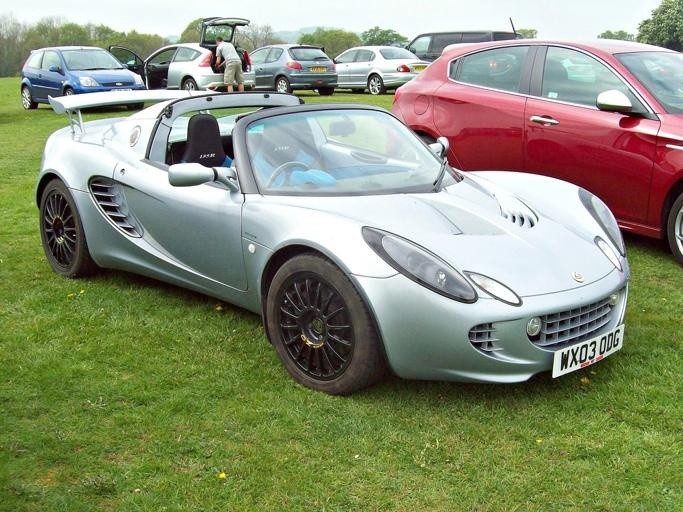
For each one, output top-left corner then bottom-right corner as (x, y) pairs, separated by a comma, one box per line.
(403, 30), (523, 63)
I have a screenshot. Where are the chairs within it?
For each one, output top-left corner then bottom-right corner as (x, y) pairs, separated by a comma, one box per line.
(180, 113), (232, 167)
(544, 60), (568, 92)
(252, 124), (321, 187)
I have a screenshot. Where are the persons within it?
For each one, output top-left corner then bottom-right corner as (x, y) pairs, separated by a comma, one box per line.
(214, 36), (244, 92)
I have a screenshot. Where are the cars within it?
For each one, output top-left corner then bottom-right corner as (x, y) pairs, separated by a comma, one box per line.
(108, 16), (255, 91)
(312, 45), (431, 95)
(388, 36), (681, 267)
(247, 43), (339, 96)
(20, 45), (145, 114)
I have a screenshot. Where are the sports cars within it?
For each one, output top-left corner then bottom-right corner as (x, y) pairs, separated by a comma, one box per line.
(35, 90), (631, 397)
